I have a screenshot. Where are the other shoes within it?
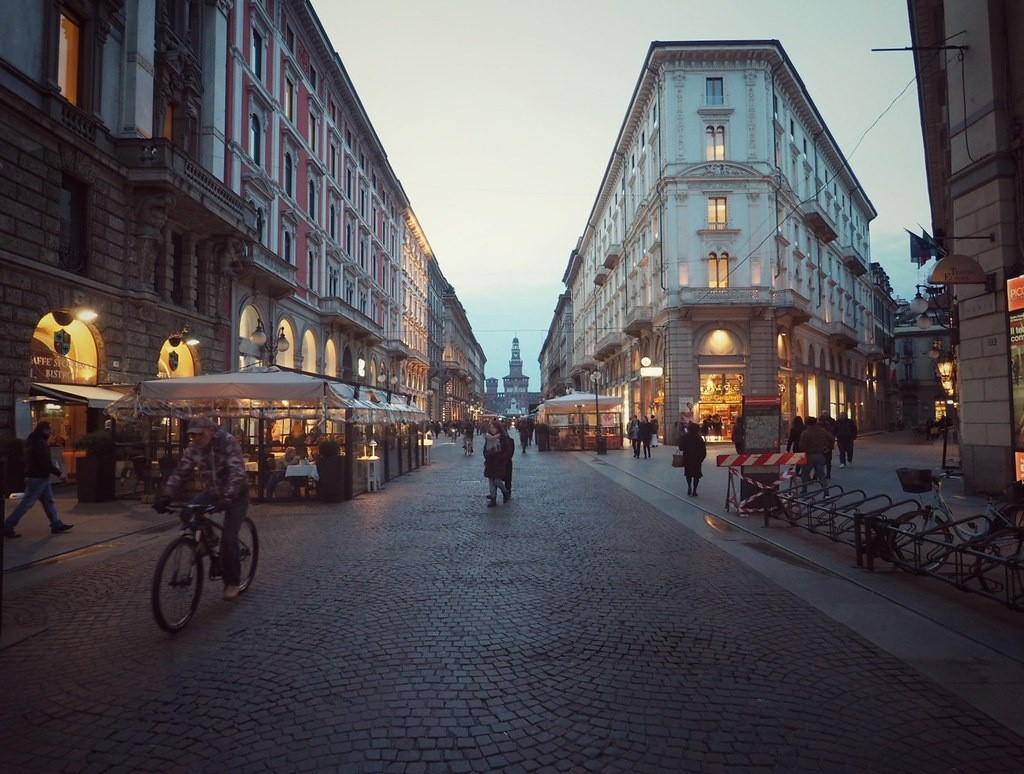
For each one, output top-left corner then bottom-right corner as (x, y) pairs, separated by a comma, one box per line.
(197, 541), (212, 555)
(825, 475), (831, 479)
(487, 495), (492, 499)
(840, 463), (844, 468)
(822, 494), (830, 501)
(3, 525), (21, 538)
(634, 454), (636, 458)
(848, 462), (854, 467)
(51, 524), (72, 534)
(687, 487), (692, 495)
(648, 453), (650, 457)
(488, 497), (497, 506)
(223, 584), (240, 599)
(693, 492), (698, 497)
(503, 490), (510, 503)
(644, 455), (647, 458)
(637, 454), (639, 458)
(800, 491), (807, 496)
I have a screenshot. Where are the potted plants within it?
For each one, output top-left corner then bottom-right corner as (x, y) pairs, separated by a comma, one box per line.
(71, 429), (113, 502)
(316, 440), (346, 503)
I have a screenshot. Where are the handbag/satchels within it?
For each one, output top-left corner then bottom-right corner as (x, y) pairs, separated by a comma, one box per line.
(931, 427), (938, 434)
(671, 449), (684, 467)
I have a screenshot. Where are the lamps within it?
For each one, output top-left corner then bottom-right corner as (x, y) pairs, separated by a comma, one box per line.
(368, 439), (380, 461)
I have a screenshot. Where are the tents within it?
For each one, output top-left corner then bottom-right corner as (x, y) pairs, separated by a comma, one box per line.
(537, 391), (623, 451)
(104, 366), (424, 498)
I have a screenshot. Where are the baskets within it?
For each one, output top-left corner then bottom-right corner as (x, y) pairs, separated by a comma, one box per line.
(896, 467), (933, 493)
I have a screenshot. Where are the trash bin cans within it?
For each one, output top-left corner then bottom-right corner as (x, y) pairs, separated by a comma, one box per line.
(597, 436), (607, 456)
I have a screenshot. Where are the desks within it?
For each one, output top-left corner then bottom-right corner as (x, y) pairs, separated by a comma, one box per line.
(244, 462), (258, 494)
(285, 465), (319, 497)
(367, 459), (380, 492)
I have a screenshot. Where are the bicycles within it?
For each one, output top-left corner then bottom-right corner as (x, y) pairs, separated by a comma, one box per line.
(150, 502), (259, 634)
(892, 469), (1024, 574)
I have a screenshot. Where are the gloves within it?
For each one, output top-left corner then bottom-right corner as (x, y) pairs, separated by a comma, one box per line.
(153, 496), (168, 513)
(216, 500), (232, 511)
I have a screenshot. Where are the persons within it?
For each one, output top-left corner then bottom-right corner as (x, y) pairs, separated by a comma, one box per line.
(430, 417), (535, 454)
(389, 424), (398, 438)
(835, 412), (857, 468)
(701, 414), (723, 436)
(786, 410), (835, 499)
(686, 402), (693, 412)
(264, 420), (338, 504)
(4, 421), (74, 539)
(484, 420), (513, 508)
(154, 416), (249, 601)
(650, 415), (658, 447)
(571, 425), (581, 447)
(731, 416), (743, 454)
(232, 423), (250, 455)
(677, 416), (706, 497)
(925, 414), (953, 440)
(627, 415), (642, 458)
(483, 422), (514, 499)
(639, 416), (653, 459)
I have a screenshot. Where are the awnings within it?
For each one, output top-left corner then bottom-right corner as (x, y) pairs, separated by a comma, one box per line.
(31, 382), (134, 408)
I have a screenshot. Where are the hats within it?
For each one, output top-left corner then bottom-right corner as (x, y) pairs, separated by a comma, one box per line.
(186, 416), (213, 433)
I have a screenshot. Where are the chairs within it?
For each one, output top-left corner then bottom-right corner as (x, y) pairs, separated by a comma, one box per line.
(266, 457), (292, 497)
(133, 455), (162, 495)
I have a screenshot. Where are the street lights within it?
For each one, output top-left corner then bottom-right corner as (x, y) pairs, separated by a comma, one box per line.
(378, 368), (398, 390)
(589, 365), (601, 449)
(249, 318), (290, 502)
